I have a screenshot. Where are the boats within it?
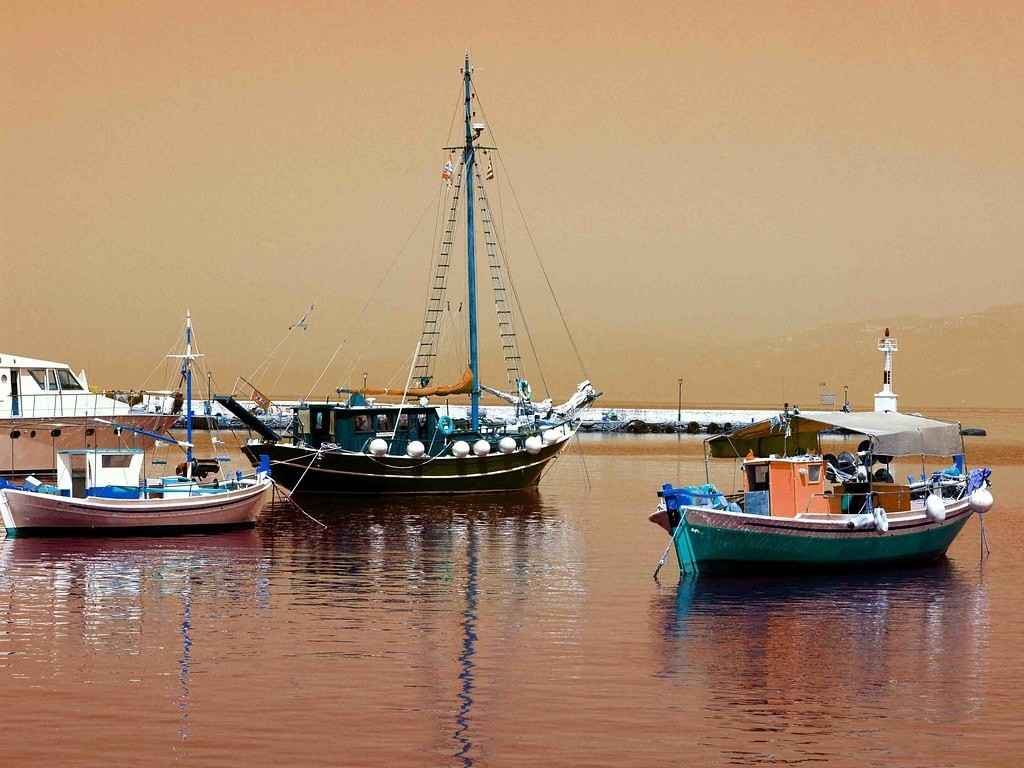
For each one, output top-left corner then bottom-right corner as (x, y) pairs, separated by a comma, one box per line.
(647, 327), (994, 581)
(0, 352), (184, 478)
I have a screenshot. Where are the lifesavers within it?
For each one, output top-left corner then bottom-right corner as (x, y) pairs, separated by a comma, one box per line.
(438, 416), (453, 434)
(518, 379), (532, 400)
(874, 508), (889, 534)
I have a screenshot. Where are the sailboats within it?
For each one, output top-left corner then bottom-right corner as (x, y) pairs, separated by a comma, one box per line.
(0, 307), (274, 539)
(213, 47), (605, 497)
